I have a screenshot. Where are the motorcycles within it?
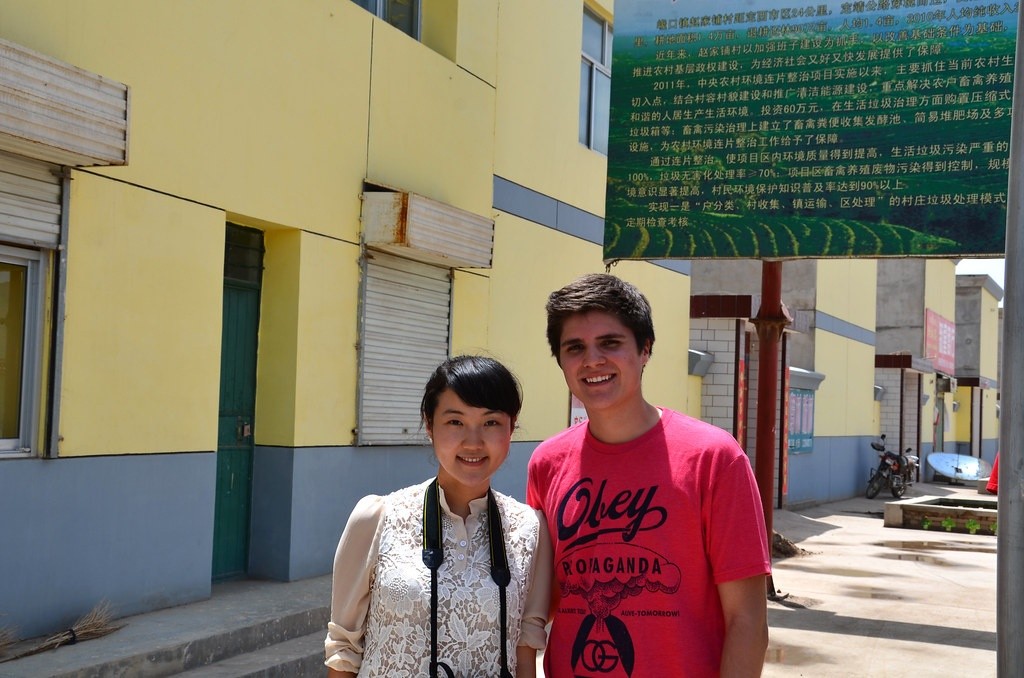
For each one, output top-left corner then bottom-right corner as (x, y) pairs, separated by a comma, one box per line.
(865, 434), (919, 500)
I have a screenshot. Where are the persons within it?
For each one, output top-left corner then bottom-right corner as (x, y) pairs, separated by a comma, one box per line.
(325, 355), (553, 678)
(525, 273), (772, 678)
